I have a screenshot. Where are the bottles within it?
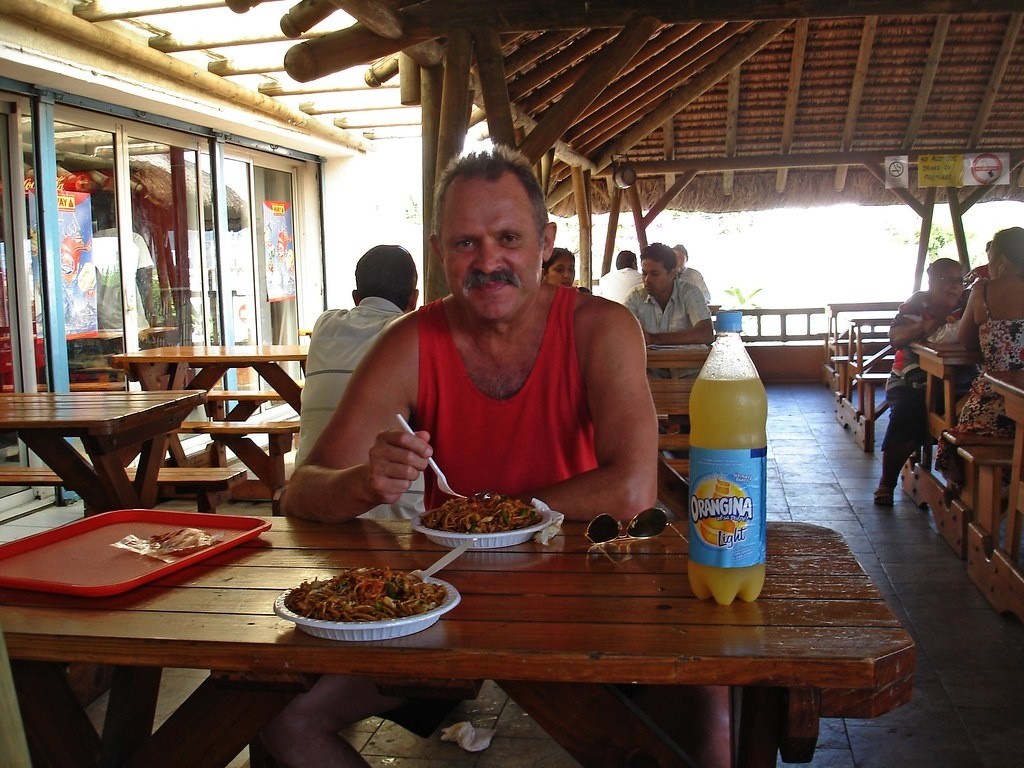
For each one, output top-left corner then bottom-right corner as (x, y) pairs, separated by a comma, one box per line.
(689, 309), (768, 606)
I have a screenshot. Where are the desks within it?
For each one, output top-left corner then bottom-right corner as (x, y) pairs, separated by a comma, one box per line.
(645, 303), (723, 477)
(67, 326), (179, 350)
(0, 389), (208, 511)
(0, 516), (919, 768)
(111, 345), (310, 469)
(819, 302), (1024, 623)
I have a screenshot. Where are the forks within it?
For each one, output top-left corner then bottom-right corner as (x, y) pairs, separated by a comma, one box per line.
(395, 413), (468, 499)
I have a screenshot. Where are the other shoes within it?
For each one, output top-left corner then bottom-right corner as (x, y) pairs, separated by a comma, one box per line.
(873, 488), (895, 506)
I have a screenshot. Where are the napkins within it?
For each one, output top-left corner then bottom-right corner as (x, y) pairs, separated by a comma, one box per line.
(531, 497), (565, 547)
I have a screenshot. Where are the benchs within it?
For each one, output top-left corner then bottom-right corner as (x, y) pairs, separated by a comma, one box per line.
(829, 338), (892, 356)
(955, 445), (1011, 548)
(943, 429), (1015, 520)
(855, 373), (891, 453)
(830, 355), (896, 394)
(2, 366), (127, 391)
(0, 391), (303, 514)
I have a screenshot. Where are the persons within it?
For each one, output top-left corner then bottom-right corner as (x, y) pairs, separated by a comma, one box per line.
(671, 244), (711, 305)
(294, 245), (426, 520)
(540, 247), (591, 296)
(936, 227), (1023, 486)
(598, 250), (645, 304)
(872, 257), (969, 506)
(622, 243), (714, 345)
(283, 153), (657, 522)
(963, 240), (992, 300)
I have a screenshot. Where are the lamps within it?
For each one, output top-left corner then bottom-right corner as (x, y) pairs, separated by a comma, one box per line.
(610, 153), (636, 189)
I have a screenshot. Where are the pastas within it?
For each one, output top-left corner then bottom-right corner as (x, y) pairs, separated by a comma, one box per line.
(287, 567), (447, 623)
(418, 491), (542, 533)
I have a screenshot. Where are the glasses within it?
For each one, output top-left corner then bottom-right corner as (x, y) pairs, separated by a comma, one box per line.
(583, 506), (691, 572)
(929, 276), (969, 286)
(586, 536), (669, 585)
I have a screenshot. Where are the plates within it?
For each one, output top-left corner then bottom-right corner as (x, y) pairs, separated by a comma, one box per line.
(274, 578), (462, 642)
(407, 509), (565, 548)
